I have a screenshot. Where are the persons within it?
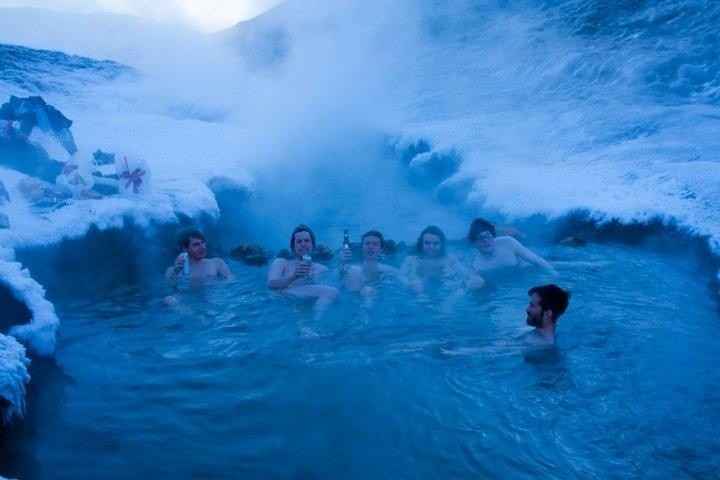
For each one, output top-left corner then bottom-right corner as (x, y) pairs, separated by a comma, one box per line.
(265, 225), (340, 314)
(161, 227), (233, 303)
(467, 217), (611, 280)
(395, 223), (472, 306)
(333, 230), (397, 320)
(440, 284), (571, 357)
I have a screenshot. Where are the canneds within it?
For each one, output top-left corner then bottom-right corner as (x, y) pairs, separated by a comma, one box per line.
(179, 252), (192, 276)
(300, 254), (314, 280)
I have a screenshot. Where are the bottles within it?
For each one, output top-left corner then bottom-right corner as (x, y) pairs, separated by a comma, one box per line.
(301, 251), (311, 280)
(341, 230), (352, 272)
